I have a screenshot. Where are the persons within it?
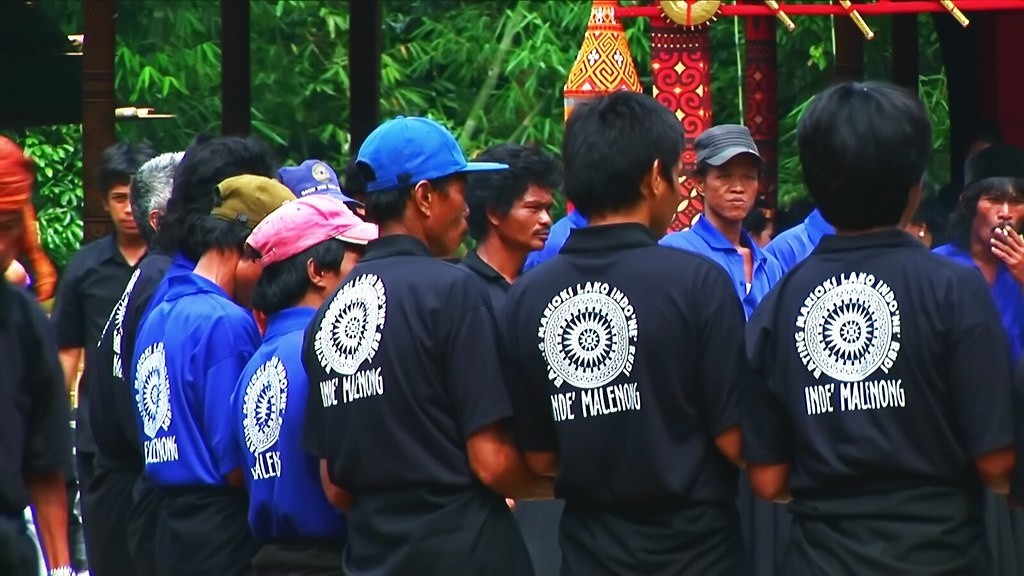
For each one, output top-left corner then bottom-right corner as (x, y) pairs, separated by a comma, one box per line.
(0, 124), (1024, 576)
(300, 116), (558, 576)
(502, 90), (752, 576)
(739, 80), (1024, 576)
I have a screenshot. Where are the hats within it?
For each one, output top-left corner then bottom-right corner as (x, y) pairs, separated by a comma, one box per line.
(210, 174), (298, 228)
(0, 135), (58, 301)
(245, 194), (379, 269)
(693, 124), (765, 166)
(356, 114), (510, 193)
(963, 145), (1024, 184)
(279, 159), (365, 209)
